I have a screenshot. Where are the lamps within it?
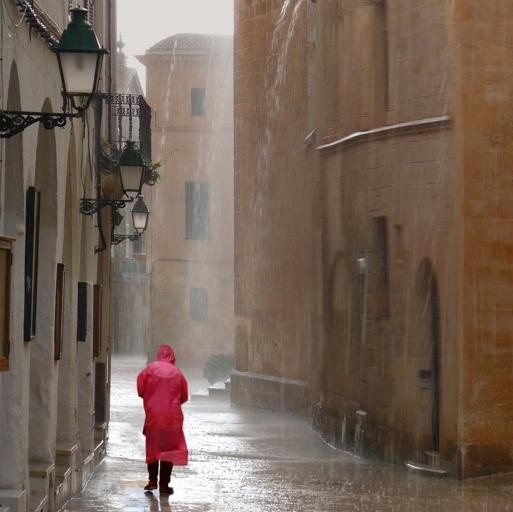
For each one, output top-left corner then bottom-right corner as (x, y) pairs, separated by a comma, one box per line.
(109, 195), (150, 249)
(74, 136), (146, 217)
(0, 1), (111, 141)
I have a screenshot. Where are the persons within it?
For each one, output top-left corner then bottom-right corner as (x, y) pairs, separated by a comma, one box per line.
(137, 345), (188, 494)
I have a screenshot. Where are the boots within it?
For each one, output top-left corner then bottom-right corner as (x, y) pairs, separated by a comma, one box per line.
(144, 476), (157, 490)
(159, 483), (173, 495)
